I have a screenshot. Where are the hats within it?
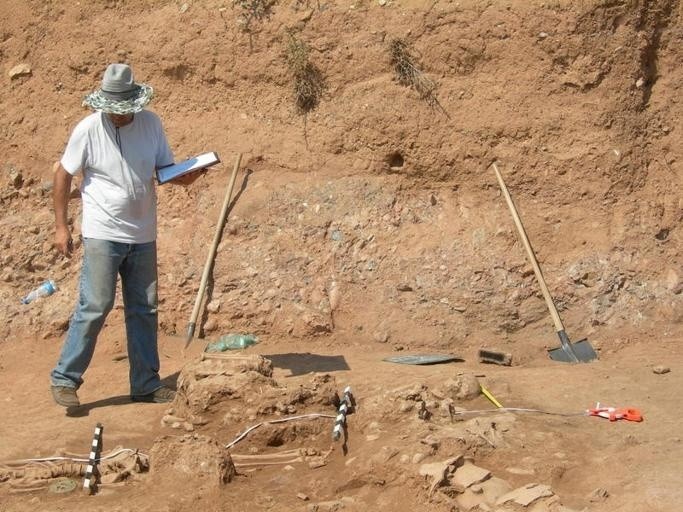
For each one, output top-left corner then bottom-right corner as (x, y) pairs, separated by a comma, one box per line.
(82, 63), (153, 114)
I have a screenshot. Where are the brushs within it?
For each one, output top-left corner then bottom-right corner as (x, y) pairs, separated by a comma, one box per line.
(478, 349), (513, 366)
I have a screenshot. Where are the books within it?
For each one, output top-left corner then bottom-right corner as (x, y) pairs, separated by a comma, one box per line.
(154, 151), (220, 185)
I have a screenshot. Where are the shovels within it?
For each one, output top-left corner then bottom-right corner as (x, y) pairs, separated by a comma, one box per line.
(493, 162), (598, 364)
(162, 151), (244, 360)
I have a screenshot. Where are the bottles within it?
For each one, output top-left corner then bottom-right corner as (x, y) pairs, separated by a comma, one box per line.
(22, 279), (56, 304)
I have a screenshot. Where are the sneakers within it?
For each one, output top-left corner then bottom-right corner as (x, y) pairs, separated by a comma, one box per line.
(50, 385), (80, 407)
(130, 387), (177, 402)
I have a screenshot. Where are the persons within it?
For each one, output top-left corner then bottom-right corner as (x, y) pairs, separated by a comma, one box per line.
(46, 64), (206, 407)
